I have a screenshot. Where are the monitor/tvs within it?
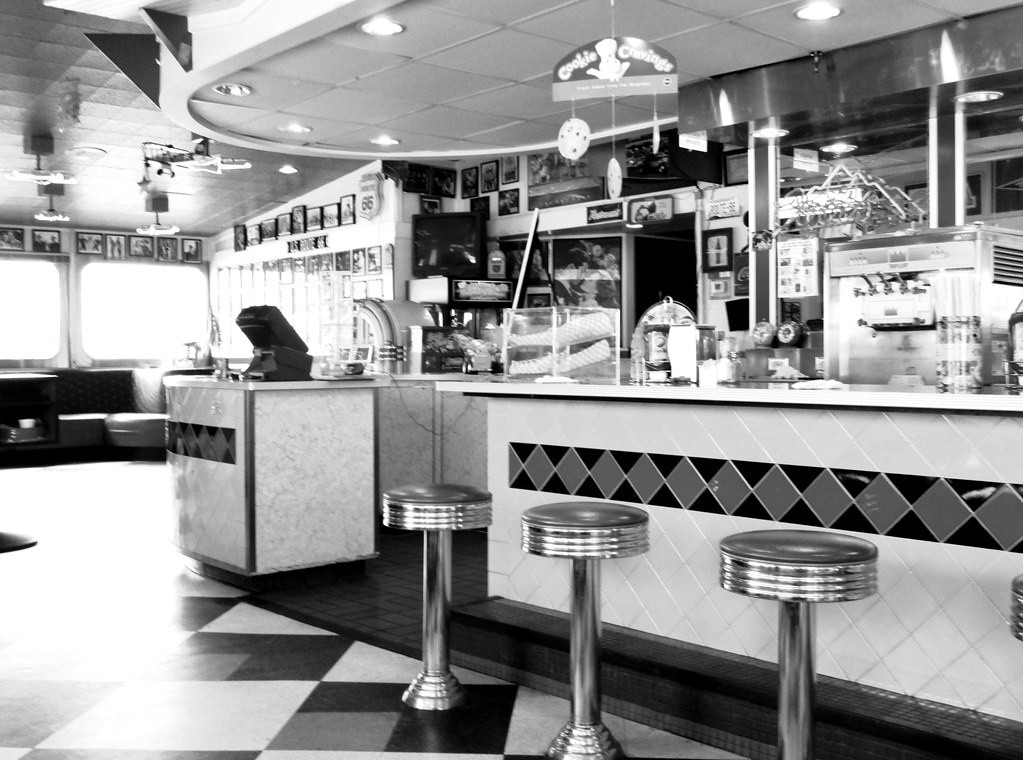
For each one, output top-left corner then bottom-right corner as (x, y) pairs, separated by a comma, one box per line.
(412, 212), (488, 280)
(236, 305), (309, 354)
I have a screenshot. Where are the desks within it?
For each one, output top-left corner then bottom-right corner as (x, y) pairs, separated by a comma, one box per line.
(0, 374), (60, 447)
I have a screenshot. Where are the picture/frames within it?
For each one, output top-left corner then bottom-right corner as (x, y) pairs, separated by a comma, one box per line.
(420, 195), (441, 214)
(904, 183), (930, 222)
(234, 195), (384, 305)
(462, 167), (478, 198)
(156, 236), (178, 261)
(966, 174), (984, 215)
(500, 155), (519, 185)
(498, 188), (519, 216)
(76, 232), (103, 255)
(31, 229), (60, 253)
(0, 227), (25, 251)
(128, 235), (154, 257)
(182, 238), (203, 264)
(703, 227), (733, 274)
(479, 159), (498, 193)
(104, 234), (126, 259)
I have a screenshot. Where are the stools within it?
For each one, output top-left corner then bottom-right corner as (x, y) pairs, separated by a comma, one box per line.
(720, 531), (878, 760)
(383, 484), (493, 710)
(519, 501), (649, 760)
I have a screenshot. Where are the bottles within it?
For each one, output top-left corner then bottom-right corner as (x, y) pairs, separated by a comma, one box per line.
(936, 316), (983, 388)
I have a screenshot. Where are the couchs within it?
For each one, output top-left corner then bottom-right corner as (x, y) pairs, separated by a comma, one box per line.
(58, 366), (188, 448)
